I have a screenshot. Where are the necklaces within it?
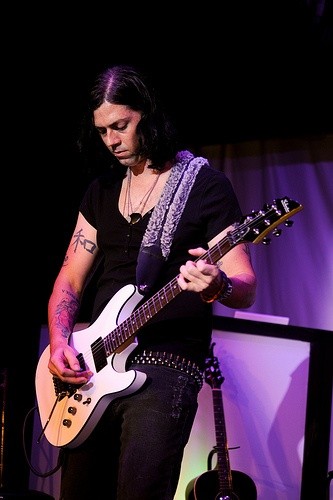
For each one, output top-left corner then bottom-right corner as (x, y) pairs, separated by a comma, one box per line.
(124, 165), (161, 225)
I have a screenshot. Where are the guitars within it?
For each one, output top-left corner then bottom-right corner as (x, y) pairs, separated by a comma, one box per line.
(34, 197), (303, 451)
(186, 343), (257, 500)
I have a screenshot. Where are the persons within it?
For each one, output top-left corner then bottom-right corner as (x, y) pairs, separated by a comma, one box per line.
(46, 66), (257, 500)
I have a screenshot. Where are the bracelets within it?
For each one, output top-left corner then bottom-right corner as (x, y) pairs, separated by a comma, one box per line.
(200, 271), (233, 303)
